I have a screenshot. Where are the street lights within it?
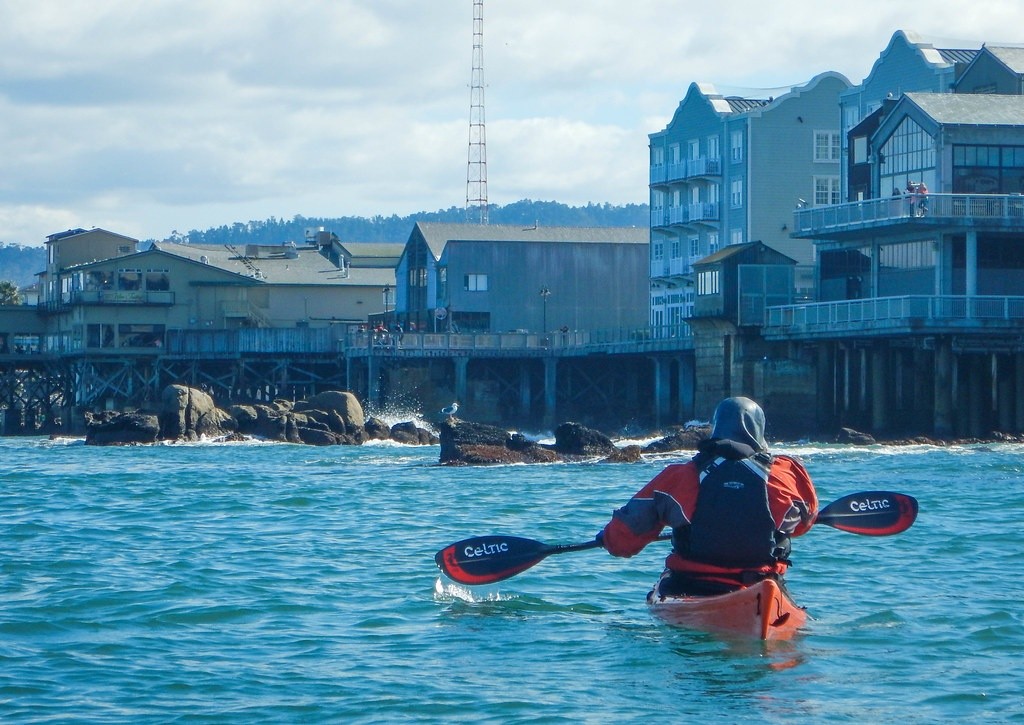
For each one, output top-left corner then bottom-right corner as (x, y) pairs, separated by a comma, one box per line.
(382, 283), (390, 331)
(539, 282), (551, 332)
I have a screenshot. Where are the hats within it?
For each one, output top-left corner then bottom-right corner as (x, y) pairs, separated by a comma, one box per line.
(710, 396), (766, 452)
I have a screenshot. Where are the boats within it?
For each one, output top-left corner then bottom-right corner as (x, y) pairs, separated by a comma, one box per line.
(432, 491), (920, 644)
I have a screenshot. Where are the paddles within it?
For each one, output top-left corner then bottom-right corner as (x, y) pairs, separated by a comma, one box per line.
(435, 491), (920, 585)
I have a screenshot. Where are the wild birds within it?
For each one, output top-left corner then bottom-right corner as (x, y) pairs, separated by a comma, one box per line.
(438, 403), (459, 419)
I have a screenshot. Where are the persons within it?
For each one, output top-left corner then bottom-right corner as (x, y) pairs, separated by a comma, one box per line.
(356, 320), (405, 345)
(903, 181), (917, 217)
(891, 186), (902, 200)
(916, 183), (929, 218)
(596, 396), (818, 604)
